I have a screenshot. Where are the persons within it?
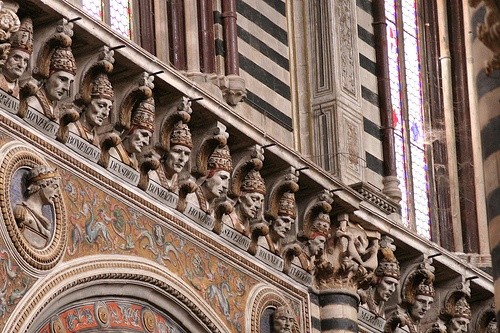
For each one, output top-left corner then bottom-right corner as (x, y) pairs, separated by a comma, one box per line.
(1, 1), (500, 333)
